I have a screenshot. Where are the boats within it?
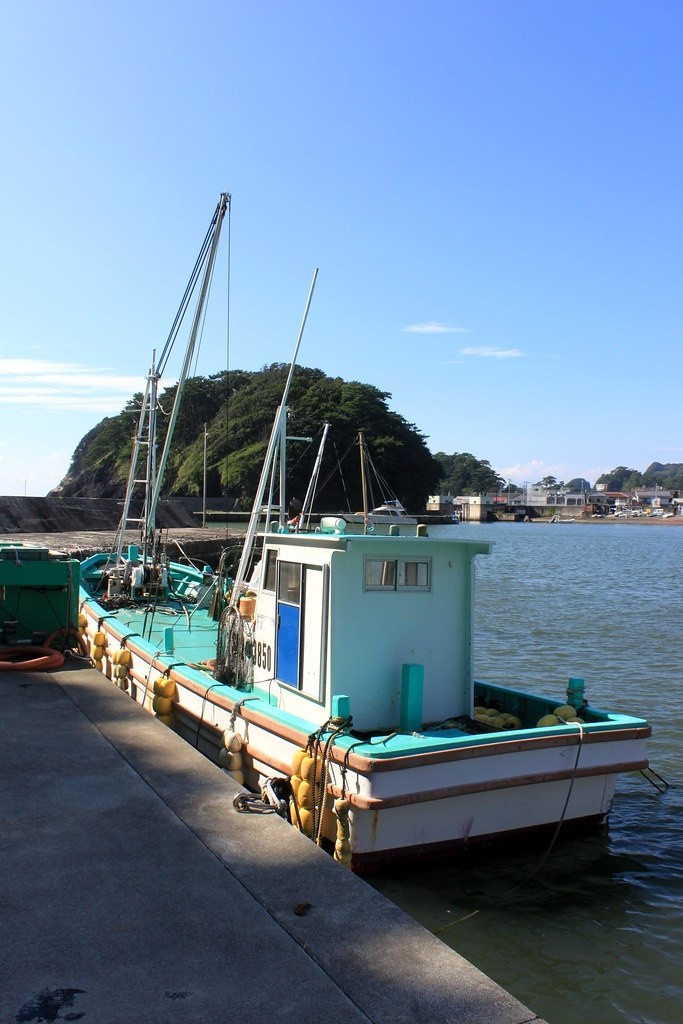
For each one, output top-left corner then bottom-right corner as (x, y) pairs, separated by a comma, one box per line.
(373, 500), (406, 515)
(79, 190), (654, 875)
(547, 504), (676, 524)
